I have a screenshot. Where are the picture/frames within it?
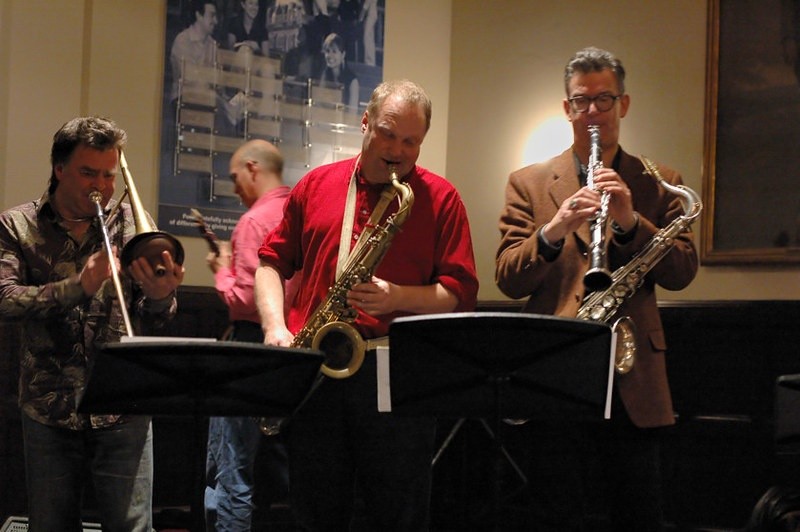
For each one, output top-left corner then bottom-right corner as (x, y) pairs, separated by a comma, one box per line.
(699, 0), (800, 270)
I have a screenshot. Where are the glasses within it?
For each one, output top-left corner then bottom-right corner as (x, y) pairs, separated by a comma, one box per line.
(568, 94), (623, 114)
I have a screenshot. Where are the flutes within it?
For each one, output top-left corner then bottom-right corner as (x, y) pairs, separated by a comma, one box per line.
(582, 125), (614, 292)
(192, 208), (220, 255)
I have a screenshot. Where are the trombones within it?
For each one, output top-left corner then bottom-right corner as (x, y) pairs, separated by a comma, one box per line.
(89, 145), (185, 339)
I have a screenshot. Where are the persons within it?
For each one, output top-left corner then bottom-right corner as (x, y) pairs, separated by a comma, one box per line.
(0, 117), (185, 532)
(204, 139), (303, 532)
(494, 47), (699, 532)
(254, 77), (480, 532)
(168, 0), (377, 150)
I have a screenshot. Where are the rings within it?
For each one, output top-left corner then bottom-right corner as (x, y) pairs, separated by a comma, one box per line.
(569, 199), (576, 205)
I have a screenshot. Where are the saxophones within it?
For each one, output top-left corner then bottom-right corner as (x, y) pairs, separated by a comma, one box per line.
(573, 153), (704, 375)
(258, 163), (415, 437)
